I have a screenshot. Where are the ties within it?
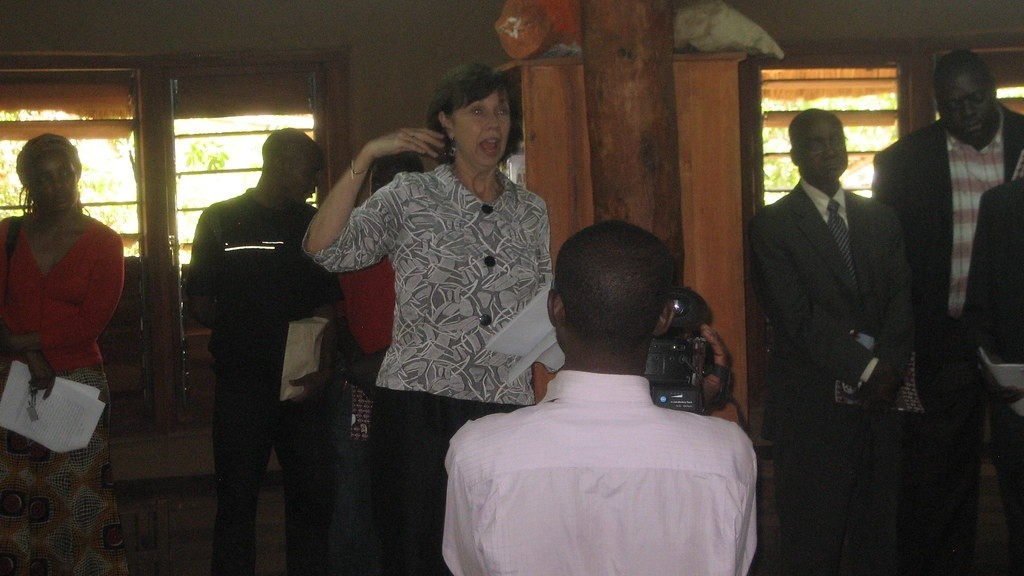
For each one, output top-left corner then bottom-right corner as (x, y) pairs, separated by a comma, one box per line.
(824, 200), (859, 297)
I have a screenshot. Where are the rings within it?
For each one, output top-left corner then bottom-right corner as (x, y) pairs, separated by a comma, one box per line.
(410, 135), (415, 142)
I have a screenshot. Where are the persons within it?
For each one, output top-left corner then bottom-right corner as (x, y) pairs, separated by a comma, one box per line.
(873, 48), (1024, 576)
(748, 109), (915, 576)
(441, 220), (758, 576)
(0, 133), (130, 576)
(188, 62), (555, 576)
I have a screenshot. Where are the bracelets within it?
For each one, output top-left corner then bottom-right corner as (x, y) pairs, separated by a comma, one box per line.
(349, 159), (367, 174)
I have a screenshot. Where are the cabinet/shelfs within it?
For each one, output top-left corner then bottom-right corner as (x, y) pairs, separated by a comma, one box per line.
(494, 52), (752, 430)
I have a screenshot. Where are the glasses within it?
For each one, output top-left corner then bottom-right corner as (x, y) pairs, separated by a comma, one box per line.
(799, 135), (849, 151)
